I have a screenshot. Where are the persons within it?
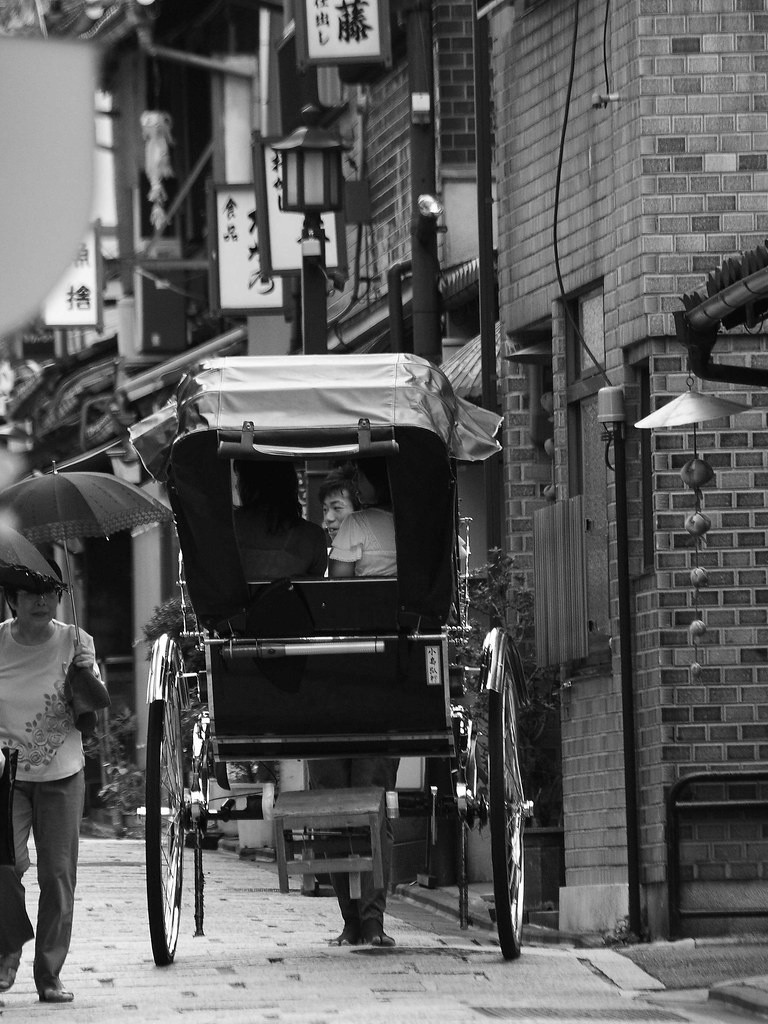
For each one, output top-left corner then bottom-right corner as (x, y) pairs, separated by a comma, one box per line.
(307, 757), (402, 946)
(318, 466), (362, 542)
(324, 448), (397, 577)
(0, 560), (111, 1003)
(232, 457), (327, 582)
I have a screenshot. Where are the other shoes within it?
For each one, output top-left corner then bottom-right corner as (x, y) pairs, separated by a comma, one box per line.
(360, 918), (395, 946)
(329, 923), (361, 947)
(0, 946), (23, 989)
(38, 982), (74, 1002)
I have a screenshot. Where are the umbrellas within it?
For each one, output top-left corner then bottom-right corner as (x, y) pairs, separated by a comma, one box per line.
(0, 523), (69, 595)
(0, 472), (175, 676)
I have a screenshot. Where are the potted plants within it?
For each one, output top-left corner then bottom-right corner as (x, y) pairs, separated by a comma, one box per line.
(131, 593), (279, 849)
(81, 703), (178, 839)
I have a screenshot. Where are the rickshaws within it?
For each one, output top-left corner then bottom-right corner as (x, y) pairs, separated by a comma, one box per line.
(140, 351), (535, 963)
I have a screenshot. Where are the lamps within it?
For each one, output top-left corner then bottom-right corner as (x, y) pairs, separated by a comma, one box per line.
(269, 102), (356, 238)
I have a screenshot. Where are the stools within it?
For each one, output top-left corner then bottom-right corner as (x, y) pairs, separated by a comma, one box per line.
(271, 786), (394, 895)
